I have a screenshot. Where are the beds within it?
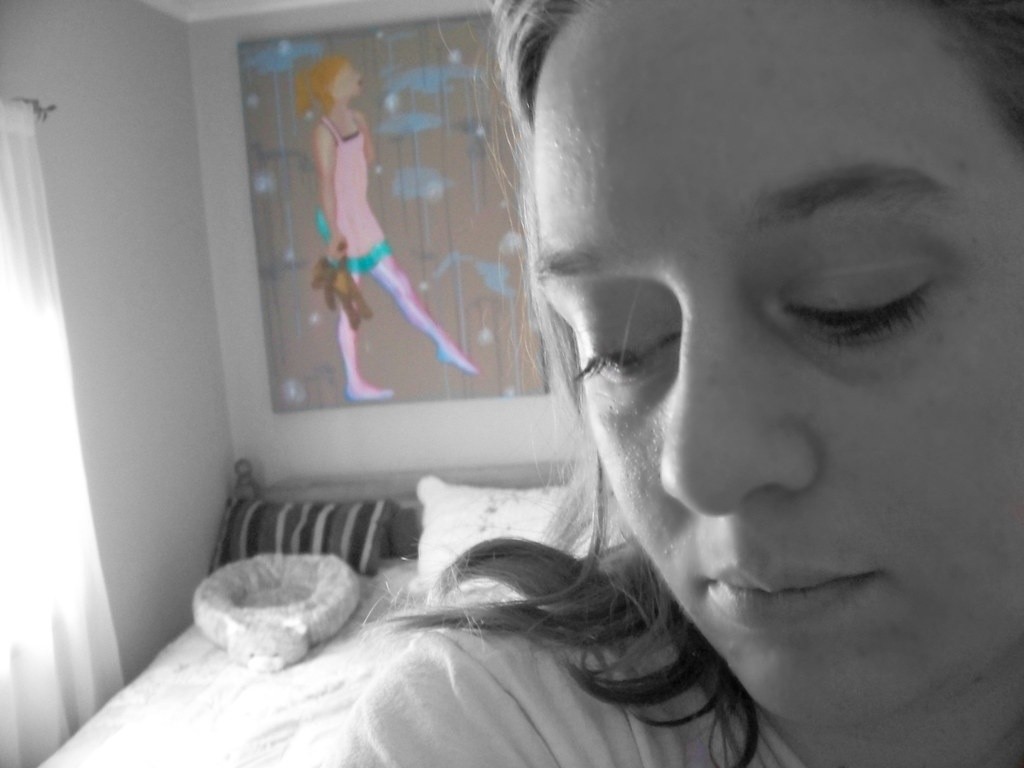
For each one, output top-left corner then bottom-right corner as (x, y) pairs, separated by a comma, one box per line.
(38, 457), (576, 768)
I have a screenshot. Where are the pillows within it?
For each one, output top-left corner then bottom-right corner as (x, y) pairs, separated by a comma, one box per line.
(208, 496), (395, 576)
(414, 476), (586, 592)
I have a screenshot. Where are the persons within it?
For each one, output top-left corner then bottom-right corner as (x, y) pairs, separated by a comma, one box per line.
(324, 0), (1024, 768)
